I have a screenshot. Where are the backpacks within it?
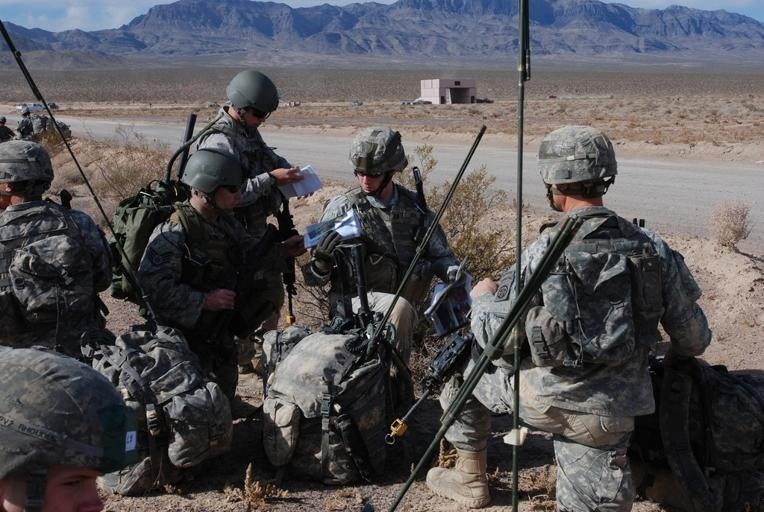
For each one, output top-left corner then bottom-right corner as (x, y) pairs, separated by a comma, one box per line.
(262, 321), (415, 488)
(79, 325), (233, 497)
(628, 358), (764, 509)
(108, 179), (238, 322)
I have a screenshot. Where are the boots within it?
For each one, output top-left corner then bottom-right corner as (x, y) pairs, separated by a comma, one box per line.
(425, 444), (491, 509)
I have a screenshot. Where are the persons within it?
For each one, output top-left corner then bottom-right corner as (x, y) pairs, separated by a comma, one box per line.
(135, 147), (309, 402)
(424, 123), (712, 512)
(0, 140), (114, 359)
(301, 127), (467, 429)
(194, 68), (306, 374)
(0, 347), (128, 512)
(0, 106), (56, 142)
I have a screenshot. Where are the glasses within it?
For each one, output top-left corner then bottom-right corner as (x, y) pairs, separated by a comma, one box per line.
(252, 109), (268, 119)
(220, 185), (240, 194)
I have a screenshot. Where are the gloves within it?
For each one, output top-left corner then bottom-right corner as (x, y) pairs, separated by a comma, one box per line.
(310, 230), (342, 279)
(441, 261), (467, 301)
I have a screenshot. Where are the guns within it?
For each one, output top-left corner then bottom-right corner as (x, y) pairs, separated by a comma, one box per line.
(335, 243), (371, 331)
(274, 202), (300, 324)
(384, 326), (475, 446)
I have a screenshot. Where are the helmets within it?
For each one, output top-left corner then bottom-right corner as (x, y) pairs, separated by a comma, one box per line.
(349, 126), (408, 175)
(180, 148), (242, 193)
(0, 348), (127, 482)
(537, 125), (617, 185)
(0, 140), (54, 183)
(226, 70), (279, 112)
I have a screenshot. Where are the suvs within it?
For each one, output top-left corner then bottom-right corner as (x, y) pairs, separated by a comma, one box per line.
(13, 102), (56, 112)
